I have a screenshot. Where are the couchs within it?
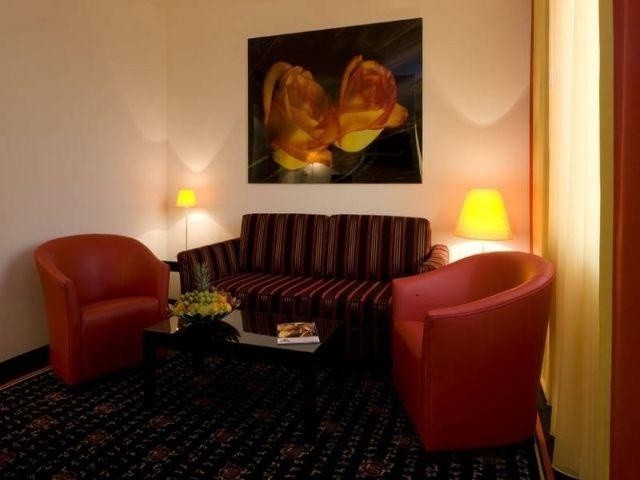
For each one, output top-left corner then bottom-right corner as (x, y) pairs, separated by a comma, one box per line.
(177, 213), (448, 356)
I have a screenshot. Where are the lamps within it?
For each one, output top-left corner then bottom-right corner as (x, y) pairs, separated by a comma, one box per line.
(176, 188), (195, 250)
(455, 186), (514, 253)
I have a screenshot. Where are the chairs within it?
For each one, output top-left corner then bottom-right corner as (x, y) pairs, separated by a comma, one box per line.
(34, 233), (170, 389)
(390, 253), (557, 451)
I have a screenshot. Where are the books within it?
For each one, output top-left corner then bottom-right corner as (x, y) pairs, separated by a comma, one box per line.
(274, 320), (321, 345)
